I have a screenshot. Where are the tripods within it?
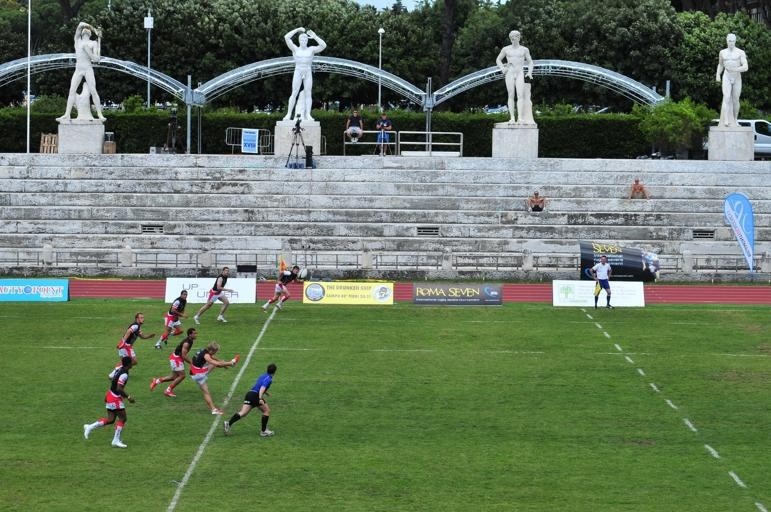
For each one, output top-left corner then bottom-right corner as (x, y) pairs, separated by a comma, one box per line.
(373, 129), (392, 156)
(284, 131), (307, 168)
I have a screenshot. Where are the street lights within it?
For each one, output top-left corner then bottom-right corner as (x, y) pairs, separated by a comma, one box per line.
(142, 8), (156, 103)
(375, 25), (388, 112)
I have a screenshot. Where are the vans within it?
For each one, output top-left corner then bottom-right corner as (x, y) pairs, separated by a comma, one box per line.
(700, 117), (770, 158)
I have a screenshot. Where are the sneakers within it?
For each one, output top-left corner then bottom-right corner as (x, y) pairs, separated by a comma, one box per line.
(84, 424), (91, 439)
(260, 429), (274, 437)
(223, 421), (231, 435)
(111, 439), (127, 448)
(109, 303), (282, 415)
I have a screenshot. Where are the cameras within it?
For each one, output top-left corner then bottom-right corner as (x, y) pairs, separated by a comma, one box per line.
(291, 119), (304, 134)
(380, 120), (388, 127)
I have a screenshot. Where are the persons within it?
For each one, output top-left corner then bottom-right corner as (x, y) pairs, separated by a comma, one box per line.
(627, 178), (650, 200)
(108, 312), (157, 380)
(60, 21), (107, 119)
(189, 341), (240, 416)
(715, 33), (749, 127)
(260, 265), (306, 312)
(154, 290), (189, 350)
(495, 30), (534, 124)
(374, 111), (392, 156)
(150, 327), (198, 398)
(83, 356), (136, 449)
(222, 364), (277, 437)
(192, 266), (234, 325)
(527, 191), (547, 212)
(346, 109), (364, 143)
(282, 26), (327, 121)
(589, 255), (614, 310)
